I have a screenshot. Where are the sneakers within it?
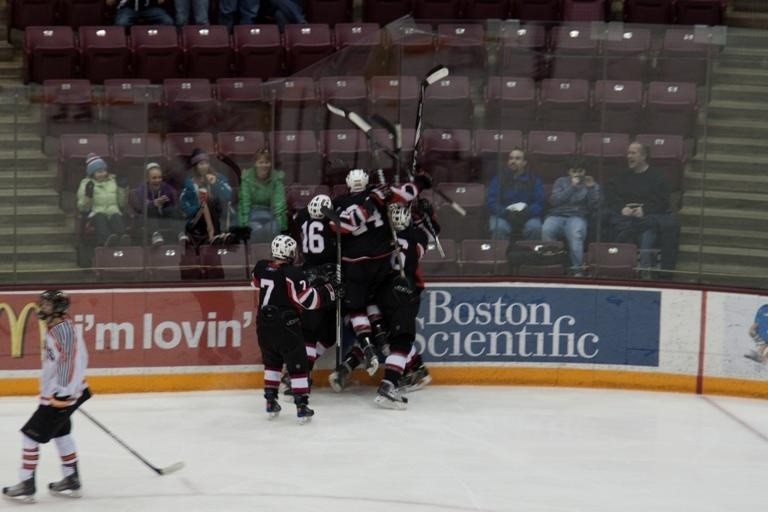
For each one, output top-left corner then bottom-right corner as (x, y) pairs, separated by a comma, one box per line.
(106, 233), (120, 247)
(177, 232), (190, 245)
(223, 233), (233, 245)
(151, 232), (164, 245)
(120, 234), (132, 247)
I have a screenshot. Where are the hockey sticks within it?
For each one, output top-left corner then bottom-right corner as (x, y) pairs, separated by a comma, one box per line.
(327, 66), (470, 286)
(78, 406), (185, 476)
(320, 204), (344, 387)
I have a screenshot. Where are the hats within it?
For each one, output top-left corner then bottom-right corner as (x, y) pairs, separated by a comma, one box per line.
(191, 148), (209, 166)
(85, 153), (106, 174)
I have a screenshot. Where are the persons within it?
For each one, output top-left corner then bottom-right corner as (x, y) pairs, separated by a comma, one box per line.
(2, 288), (91, 503)
(106, 0), (308, 26)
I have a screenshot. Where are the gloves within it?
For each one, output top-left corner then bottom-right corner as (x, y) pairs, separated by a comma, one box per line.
(327, 279), (347, 299)
(48, 392), (76, 409)
(369, 185), (391, 202)
(85, 180), (94, 197)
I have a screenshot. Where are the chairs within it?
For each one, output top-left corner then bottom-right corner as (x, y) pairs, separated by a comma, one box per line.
(14, 3), (722, 283)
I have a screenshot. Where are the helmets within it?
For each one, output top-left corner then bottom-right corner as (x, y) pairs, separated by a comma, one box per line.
(370, 168), (395, 187)
(345, 168), (369, 192)
(307, 194), (334, 220)
(38, 289), (69, 323)
(271, 235), (298, 261)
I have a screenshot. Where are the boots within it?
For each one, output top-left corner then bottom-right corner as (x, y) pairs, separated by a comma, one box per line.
(3, 469), (36, 496)
(263, 388), (282, 412)
(294, 392), (314, 418)
(376, 369), (407, 403)
(48, 463), (81, 491)
(399, 355), (427, 386)
(334, 319), (388, 390)
